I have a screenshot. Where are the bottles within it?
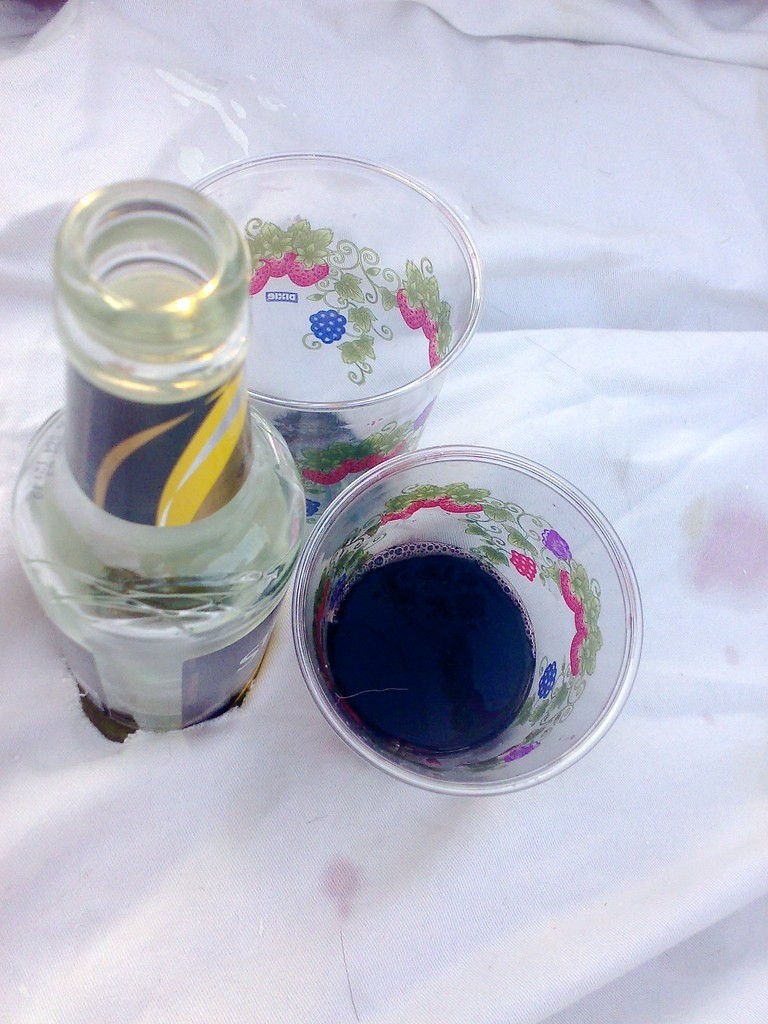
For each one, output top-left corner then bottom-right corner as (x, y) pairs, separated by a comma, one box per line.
(12, 177), (304, 730)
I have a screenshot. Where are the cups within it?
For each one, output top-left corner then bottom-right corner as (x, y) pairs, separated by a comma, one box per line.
(190, 153), (481, 520)
(292, 445), (644, 796)
